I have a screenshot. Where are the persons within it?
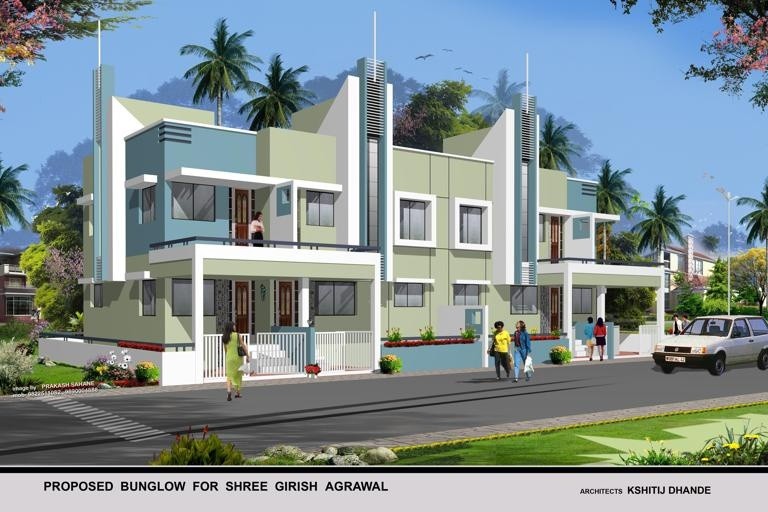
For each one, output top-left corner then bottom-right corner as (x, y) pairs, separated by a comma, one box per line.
(222, 322), (249, 401)
(682, 315), (691, 329)
(250, 212), (264, 247)
(584, 317), (595, 361)
(487, 321), (512, 380)
(673, 315), (682, 335)
(594, 318), (606, 361)
(511, 321), (531, 383)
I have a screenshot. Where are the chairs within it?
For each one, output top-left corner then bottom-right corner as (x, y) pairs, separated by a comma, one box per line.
(709, 325), (720, 333)
(734, 326), (744, 332)
